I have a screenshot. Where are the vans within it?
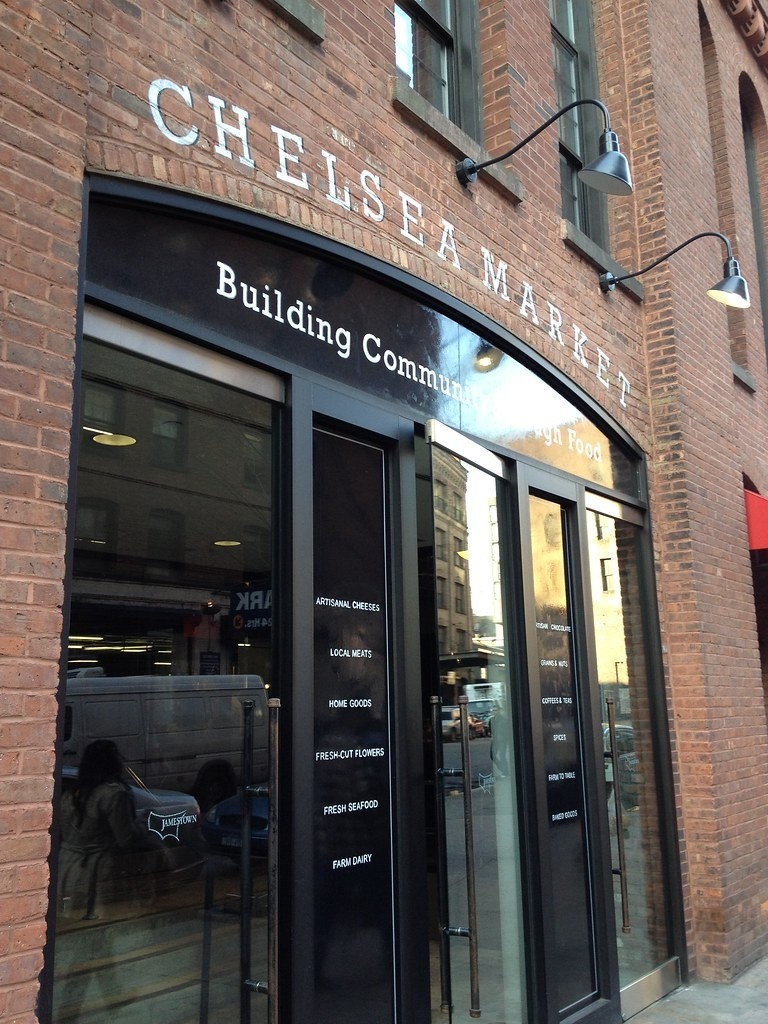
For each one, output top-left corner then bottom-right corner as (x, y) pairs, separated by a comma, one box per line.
(64, 675), (270, 785)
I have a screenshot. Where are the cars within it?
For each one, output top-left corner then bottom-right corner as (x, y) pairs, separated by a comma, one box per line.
(200, 783), (271, 859)
(61, 765), (202, 849)
(602, 723), (636, 783)
(437, 682), (503, 743)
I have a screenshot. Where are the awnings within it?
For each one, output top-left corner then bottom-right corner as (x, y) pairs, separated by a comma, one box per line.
(743, 489), (768, 551)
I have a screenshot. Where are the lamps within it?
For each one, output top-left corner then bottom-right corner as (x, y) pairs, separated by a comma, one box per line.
(456, 98), (634, 195)
(598, 231), (750, 308)
(474, 339), (505, 374)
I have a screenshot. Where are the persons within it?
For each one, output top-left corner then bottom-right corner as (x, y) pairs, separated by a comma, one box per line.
(489, 706), (510, 778)
(57, 739), (140, 1024)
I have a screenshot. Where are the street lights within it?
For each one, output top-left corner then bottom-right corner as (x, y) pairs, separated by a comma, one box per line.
(614, 661), (623, 716)
